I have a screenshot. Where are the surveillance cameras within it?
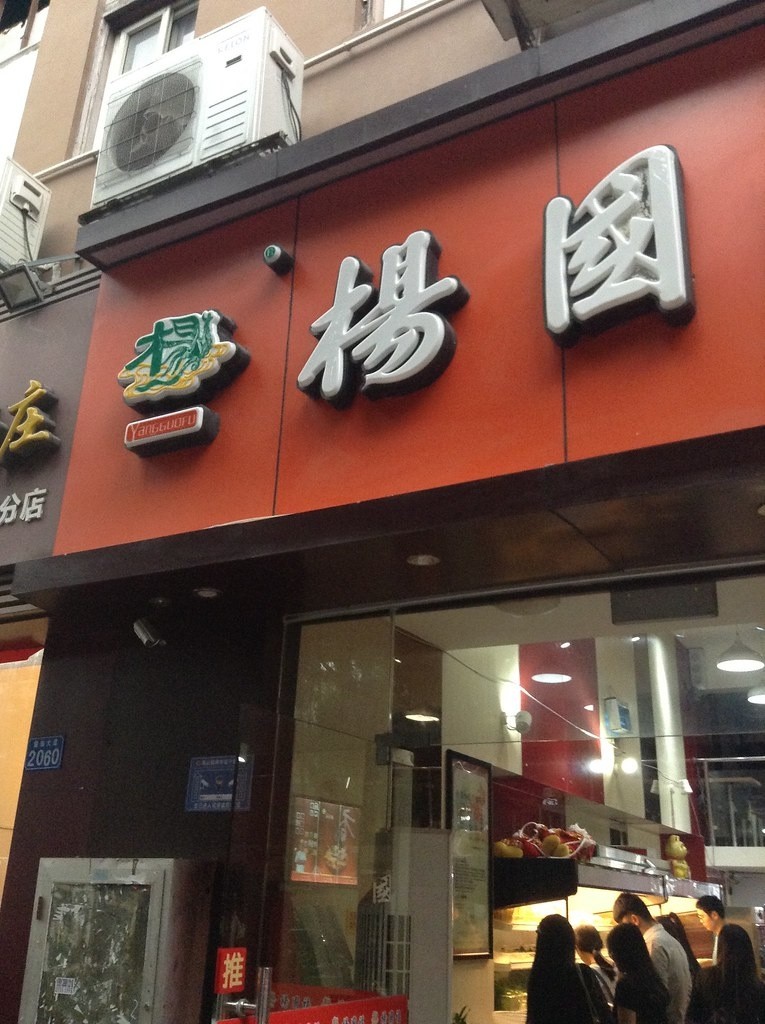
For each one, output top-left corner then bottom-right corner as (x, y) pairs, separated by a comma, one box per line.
(515, 711), (532, 734)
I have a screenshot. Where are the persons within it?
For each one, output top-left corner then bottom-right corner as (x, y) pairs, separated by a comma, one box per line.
(525, 894), (765, 1024)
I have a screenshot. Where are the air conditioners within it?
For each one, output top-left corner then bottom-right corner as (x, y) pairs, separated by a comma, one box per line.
(0, 157), (53, 278)
(94, 8), (304, 213)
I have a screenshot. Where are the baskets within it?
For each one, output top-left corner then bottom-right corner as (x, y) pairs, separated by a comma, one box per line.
(510, 822), (586, 863)
